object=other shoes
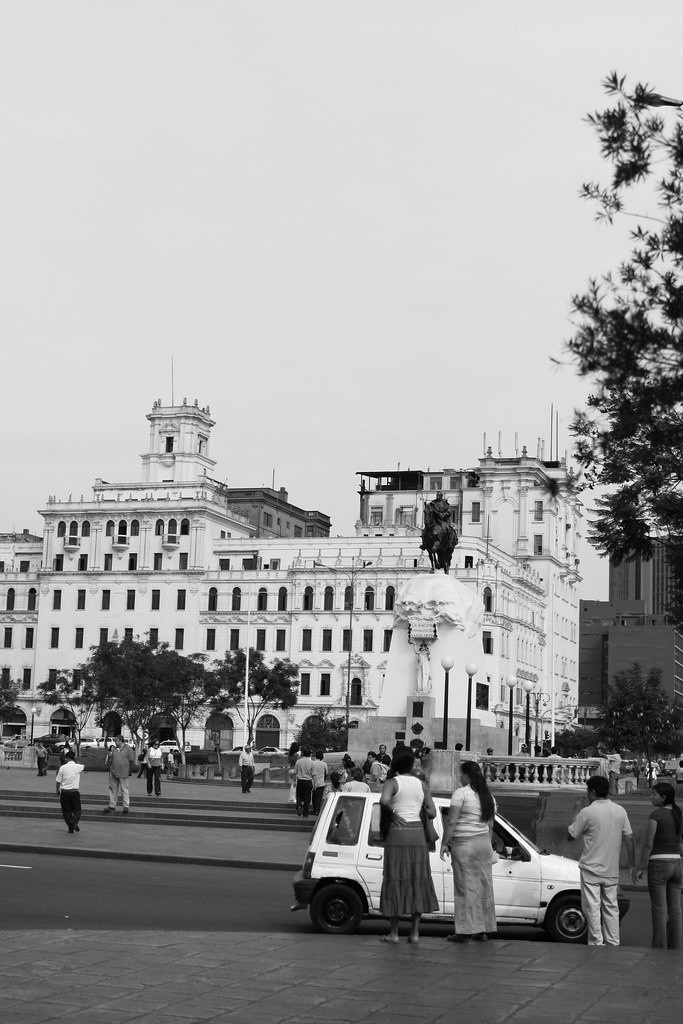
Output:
[104,806,116,811]
[472,934,488,941]
[447,933,468,942]
[71,819,80,831]
[69,830,74,834]
[123,807,129,812]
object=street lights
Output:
[466,663,478,751]
[31,707,36,745]
[506,677,516,756]
[523,680,533,750]
[314,560,373,751]
[441,656,455,750]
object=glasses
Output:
[154,744,159,745]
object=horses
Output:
[422,502,459,575]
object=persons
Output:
[487,748,495,755]
[323,745,392,802]
[455,743,463,751]
[239,745,255,794]
[167,749,174,780]
[36,742,50,776]
[145,739,164,797]
[61,744,70,765]
[288,742,328,817]
[636,782,683,950]
[137,749,148,778]
[56,751,85,833]
[633,767,640,786]
[104,736,135,813]
[567,775,637,947]
[440,761,498,942]
[419,491,451,550]
[513,743,562,783]
[378,746,439,944]
[675,760,683,784]
[105,745,116,780]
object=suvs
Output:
[55,737,98,749]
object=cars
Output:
[253,746,289,757]
[96,737,136,751]
[34,734,71,748]
[220,746,260,755]
[4,734,32,748]
[291,791,632,945]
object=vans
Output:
[159,740,191,752]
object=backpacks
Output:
[138,753,145,761]
[371,762,390,781]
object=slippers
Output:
[379,935,399,943]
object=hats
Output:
[341,756,350,760]
[245,745,250,748]
